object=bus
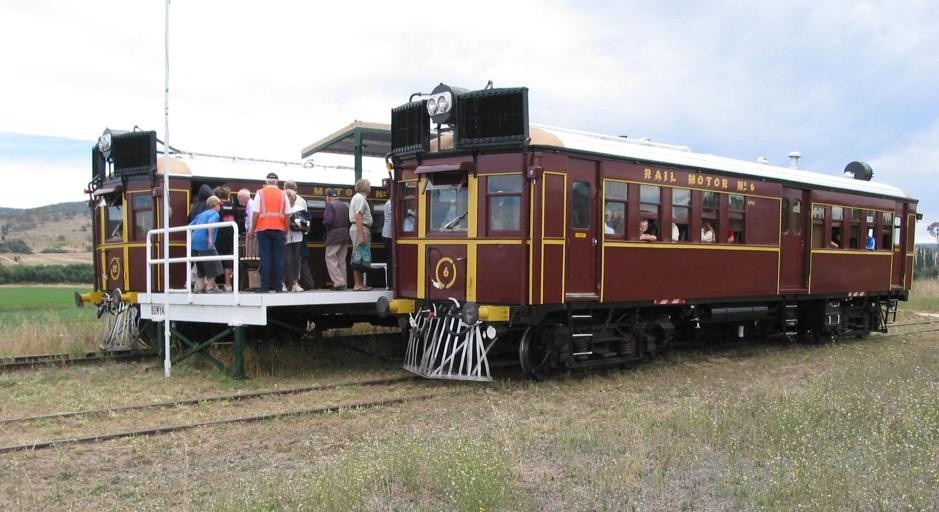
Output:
[390,76,925,384]
[75,125,396,351]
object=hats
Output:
[267,173,278,179]
[206,196,224,207]
[327,189,337,197]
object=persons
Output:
[728,226,736,242]
[831,236,841,249]
[183,170,415,295]
[700,222,716,242]
[865,228,876,250]
[639,219,658,241]
[672,220,680,241]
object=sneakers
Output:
[353,285,373,290]
[331,285,345,291]
[184,282,232,293]
[255,282,304,293]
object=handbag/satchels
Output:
[350,259,370,271]
